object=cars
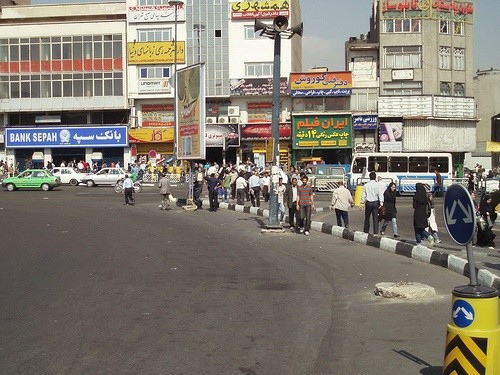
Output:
[80,167,134,188]
[37,167,93,186]
[1,169,62,192]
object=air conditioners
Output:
[129,99,136,128]
[218,117,228,123]
[228,106,240,116]
[239,111,248,124]
[205,117,216,123]
[230,117,238,123]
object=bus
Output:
[348,152,453,195]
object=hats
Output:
[131,163,136,166]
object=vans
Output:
[299,165,346,185]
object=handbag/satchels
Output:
[379,207,385,221]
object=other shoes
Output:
[290,227,294,230]
[429,236,434,244]
[436,240,442,244]
[394,234,399,237]
[132,200,135,205]
[380,230,385,234]
[300,227,304,233]
[304,231,309,235]
[488,246,494,250]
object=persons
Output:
[330,182,355,228]
[413,163,500,249]
[359,172,400,238]
[0,156,316,235]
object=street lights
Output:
[169,0,185,174]
